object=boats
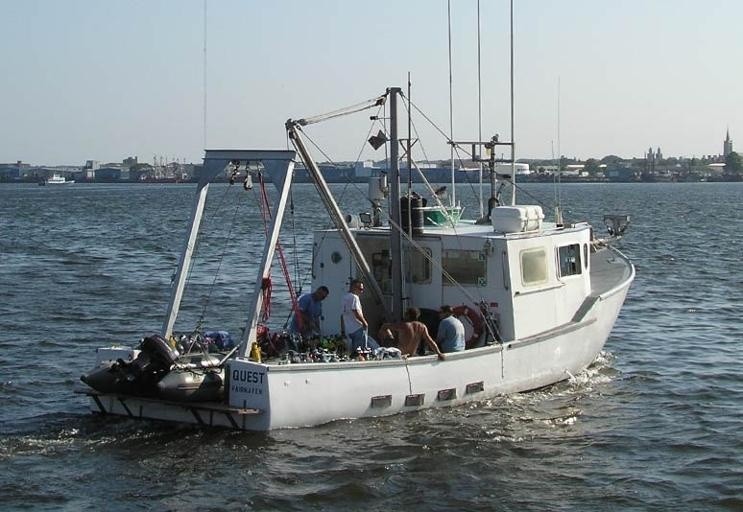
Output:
[38,171,75,187]
[74,86,635,432]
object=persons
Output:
[380,305,447,361]
[431,305,465,353]
[288,285,329,340]
[340,278,368,351]
